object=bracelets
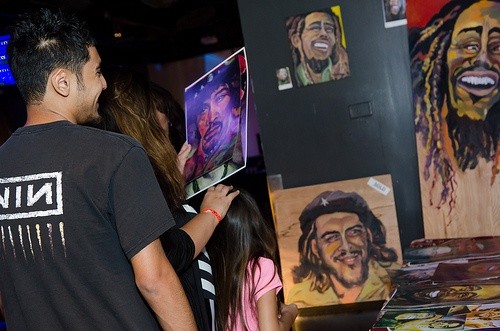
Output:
[201,208,222,222]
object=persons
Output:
[93,69,299,331]
[0,8,198,331]
